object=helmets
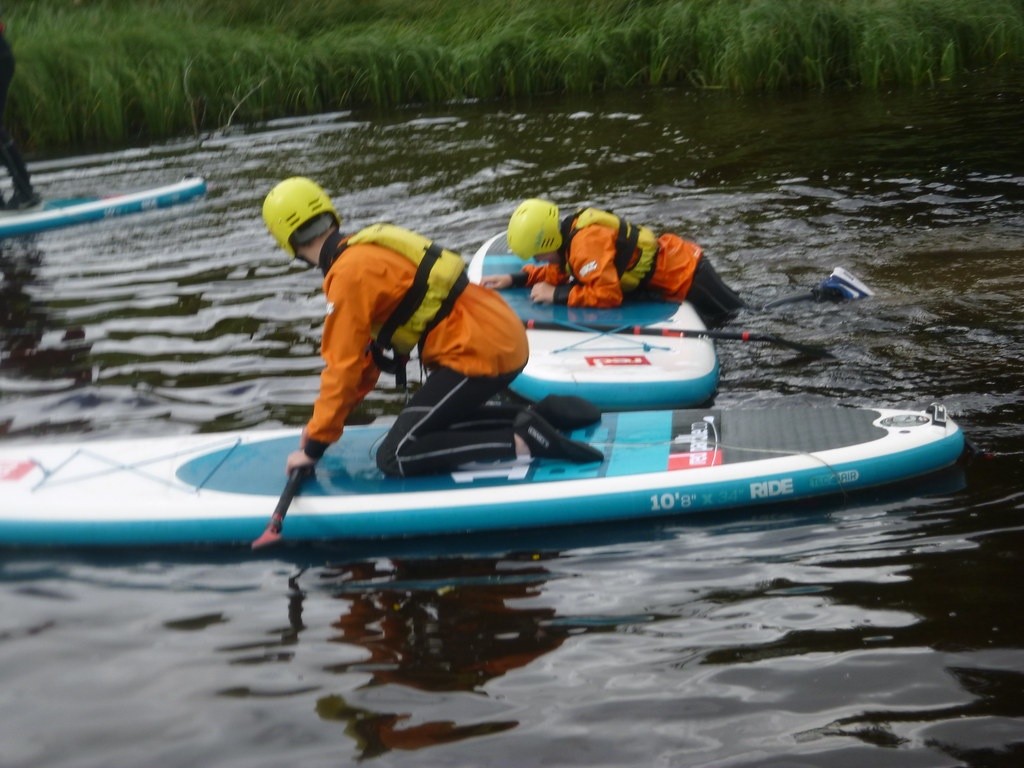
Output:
[507,199,562,261]
[263,176,341,259]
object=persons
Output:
[479,197,875,325]
[260,176,606,479]
[0,17,41,208]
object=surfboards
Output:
[0,401,964,552]
[468,230,720,412]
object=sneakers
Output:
[527,394,600,429]
[514,409,605,463]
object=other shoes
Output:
[823,267,875,300]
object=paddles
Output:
[249,465,301,552]
[522,318,819,352]
